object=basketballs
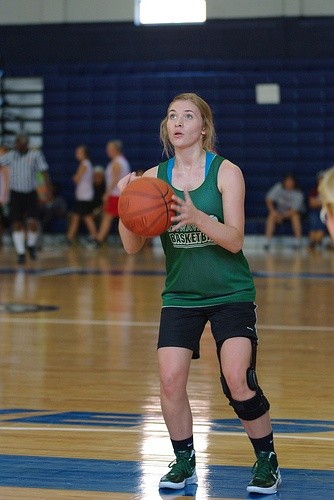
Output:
[118,177,178,237]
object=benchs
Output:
[0,58,334,232]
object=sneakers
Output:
[159,450,198,489]
[247,451,282,494]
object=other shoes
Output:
[87,238,101,247]
[56,239,71,248]
[28,252,37,260]
[16,256,26,264]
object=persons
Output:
[63,138,130,248]
[0,134,62,265]
[263,176,305,252]
[306,167,334,262]
[118,93,282,494]
